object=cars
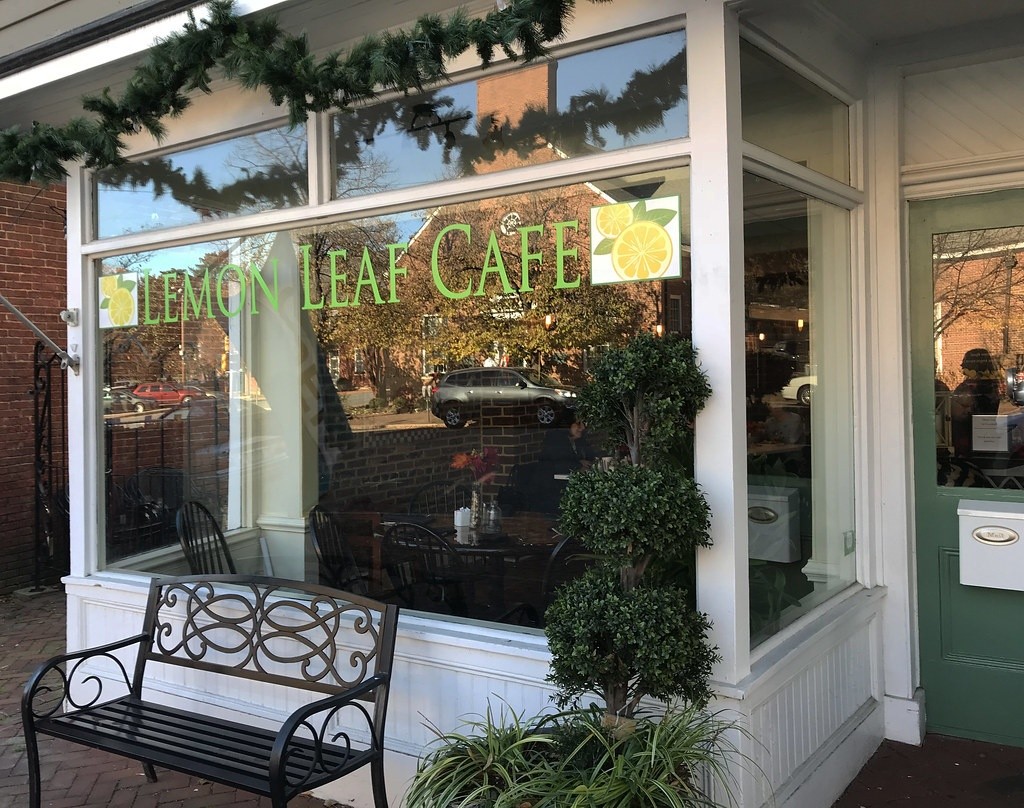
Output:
[782,375,817,406]
[332,376,352,392]
[102,378,270,427]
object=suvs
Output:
[1005,353,1024,407]
[431,367,586,429]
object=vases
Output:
[468,482,482,530]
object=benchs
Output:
[20,575,406,808]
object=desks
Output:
[747,443,805,478]
[380,510,569,621]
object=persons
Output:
[529,407,601,513]
[748,382,806,479]
[950,348,1000,488]
[933,358,952,487]
[937,379,992,488]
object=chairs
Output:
[304,463,583,620]
[175,499,239,576]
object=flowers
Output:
[449,448,499,488]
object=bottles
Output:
[490,501,502,533]
[480,503,489,533]
